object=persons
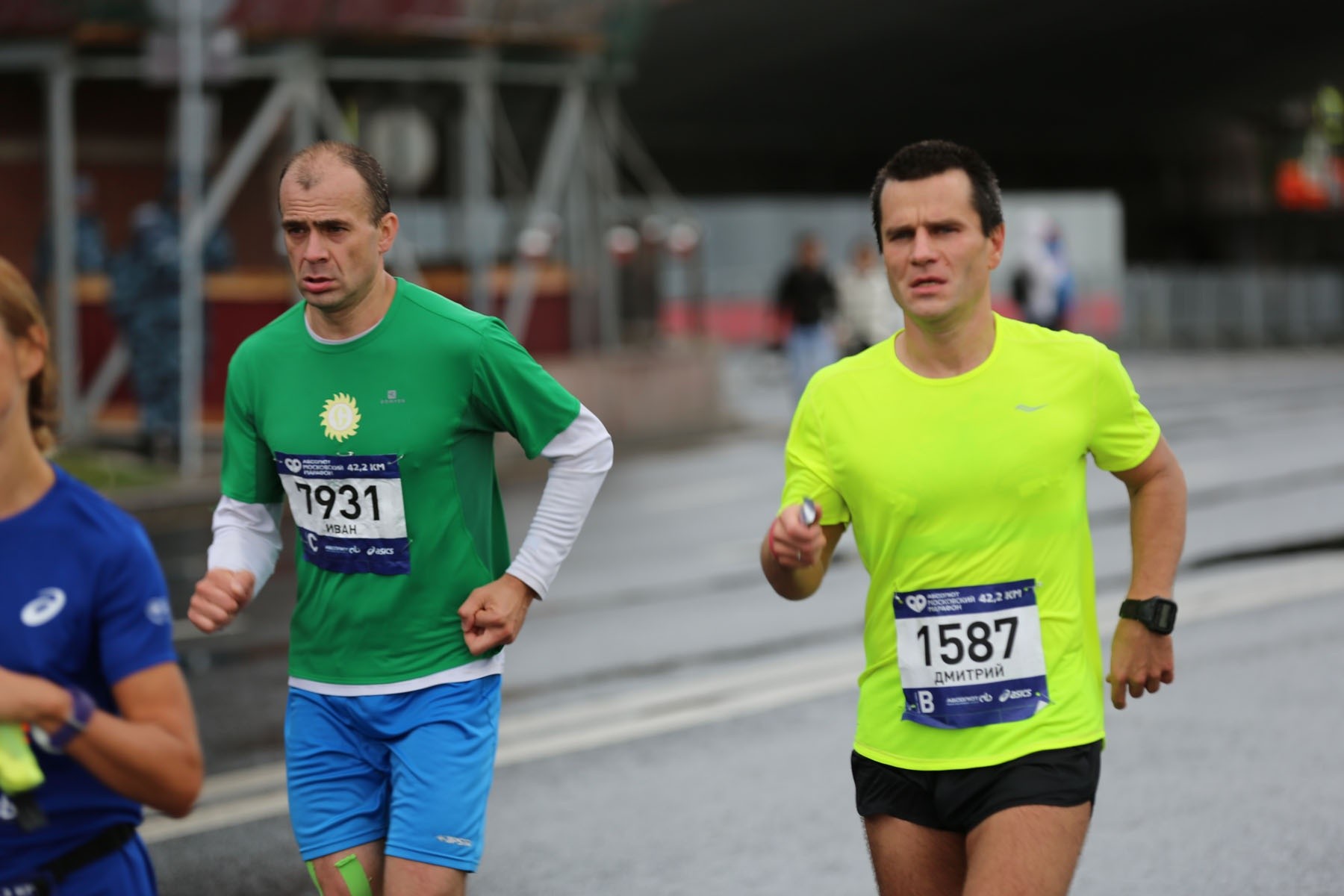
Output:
[20,166,207,467]
[186,143,615,895]
[760,138,1186,896]
[0,250,203,896]
[771,202,1070,406]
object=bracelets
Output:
[769,518,778,562]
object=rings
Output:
[797,549,805,565]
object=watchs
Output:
[32,686,93,757]
[1119,596,1178,637]
